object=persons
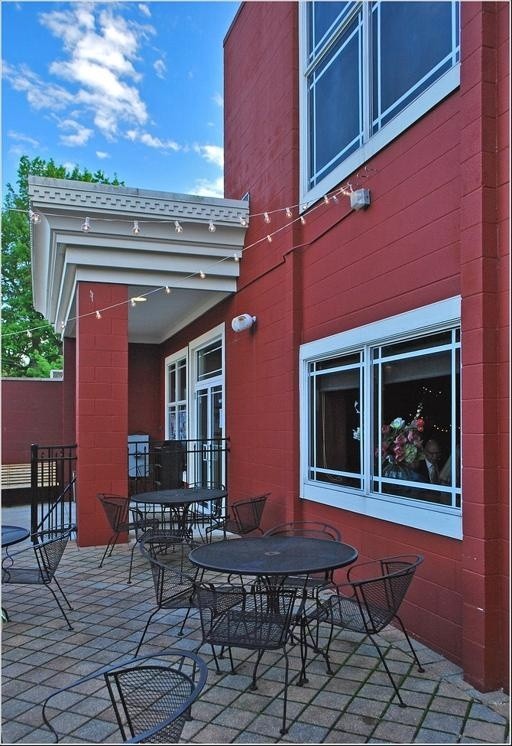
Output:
[415,438,445,502]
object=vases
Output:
[382,461,414,493]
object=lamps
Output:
[231,314,257,334]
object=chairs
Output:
[314,553,425,709]
[41,650,208,745]
[206,491,272,553]
[1,523,76,631]
[95,492,160,583]
[252,519,343,644]
[131,534,246,675]
[186,580,333,735]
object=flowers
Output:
[350,401,428,468]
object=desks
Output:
[131,488,229,551]
[0,524,31,622]
[189,535,360,691]
